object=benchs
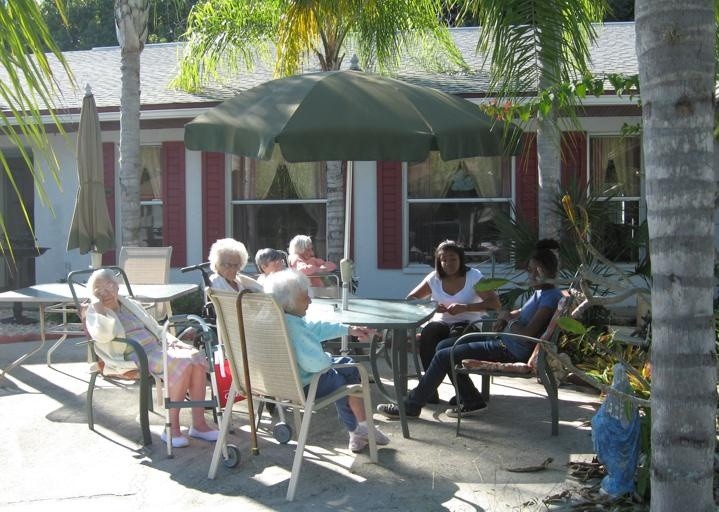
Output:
[605,295,651,347]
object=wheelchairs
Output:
[273,248,364,363]
[179,260,221,410]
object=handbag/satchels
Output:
[212,359,247,408]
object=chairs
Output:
[382,251,500,402]
[77,246,177,364]
[204,286,378,502]
[450,289,571,437]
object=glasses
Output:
[223,263,240,269]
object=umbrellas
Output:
[67,83,116,275]
[183,52,526,359]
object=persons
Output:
[206,236,275,413]
[286,234,358,298]
[265,267,390,451]
[419,194,486,263]
[401,239,501,404]
[376,237,566,419]
[253,248,284,285]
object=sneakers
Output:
[446,402,488,417]
[189,424,220,441]
[349,422,389,452]
[160,430,189,447]
[377,404,420,419]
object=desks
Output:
[302,296,439,438]
[0,283,199,387]
